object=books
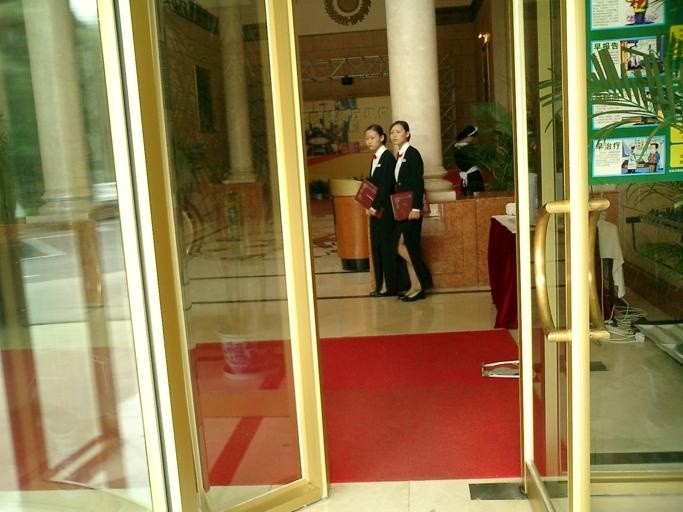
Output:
[389,189,431,222]
[355,178,385,219]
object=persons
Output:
[453,126,485,196]
[628,147,637,173]
[631,0,649,25]
[389,120,433,301]
[628,45,642,70]
[364,125,397,297]
[648,143,660,172]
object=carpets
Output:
[0,328,573,491]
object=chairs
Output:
[167,130,268,262]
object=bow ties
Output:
[397,152,402,160]
[372,154,376,159]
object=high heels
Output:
[370,283,425,304]
